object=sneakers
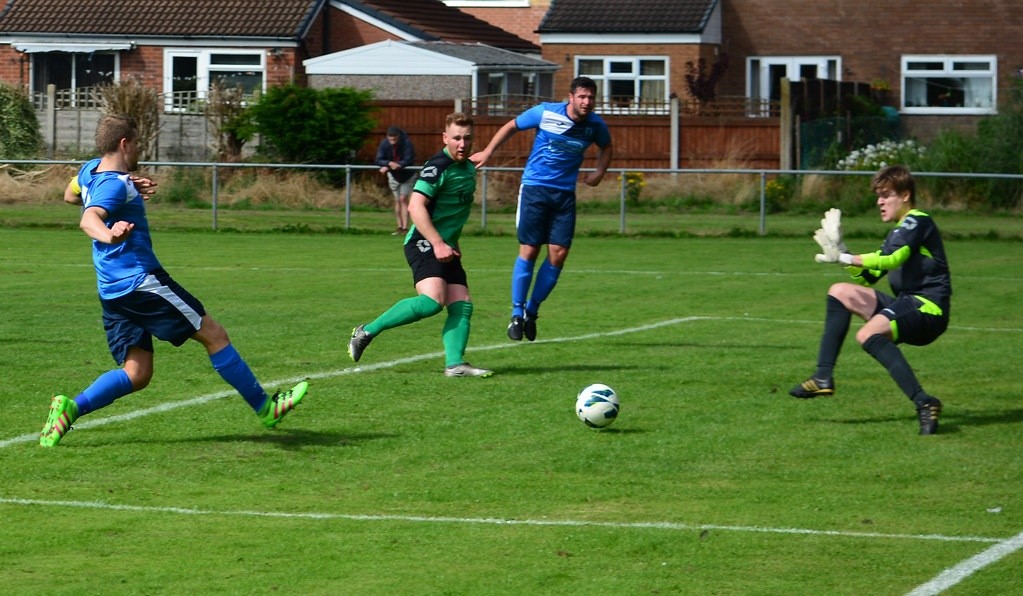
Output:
[347,325,372,363]
[38,394,76,447]
[444,362,495,378]
[790,376,835,398]
[916,397,942,434]
[507,317,522,339]
[260,381,307,428]
[524,308,538,341]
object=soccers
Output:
[575,383,619,428]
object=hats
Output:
[388,126,398,135]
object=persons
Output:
[345,111,496,378]
[789,167,954,435]
[467,78,613,342]
[377,126,419,235]
[39,113,310,452]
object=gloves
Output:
[821,207,848,254]
[813,228,840,264]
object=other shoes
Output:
[391,227,407,237]
